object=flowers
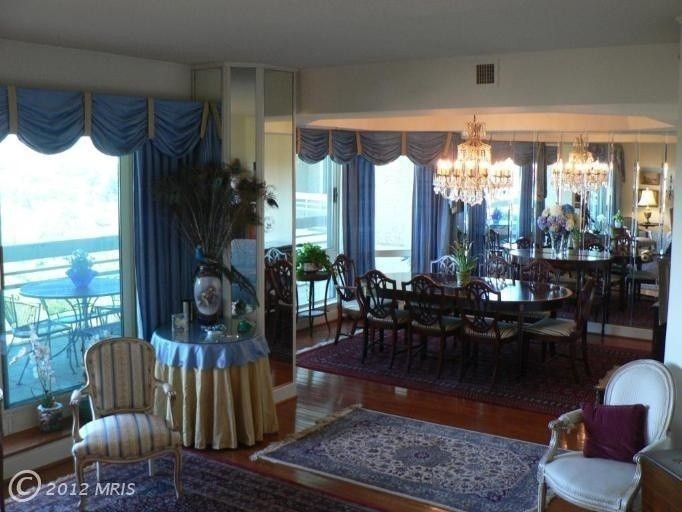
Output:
[537,202,578,249]
[64,249,96,272]
[9,324,57,408]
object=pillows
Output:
[578,401,649,465]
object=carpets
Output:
[4,448,385,512]
[249,404,578,512]
[297,317,657,416]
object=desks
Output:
[633,448,682,512]
[155,322,263,450]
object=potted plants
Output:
[613,209,624,229]
[295,243,332,281]
[437,223,484,288]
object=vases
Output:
[193,263,222,329]
[37,402,64,434]
[65,269,98,289]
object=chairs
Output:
[485,217,667,325]
[536,359,676,512]
[4,276,121,385]
[68,337,184,512]
[330,254,605,389]
[264,248,292,349]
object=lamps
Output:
[432,112,513,207]
[638,187,659,224]
[552,134,609,196]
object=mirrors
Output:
[191,62,296,405]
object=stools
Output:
[296,269,331,338]
